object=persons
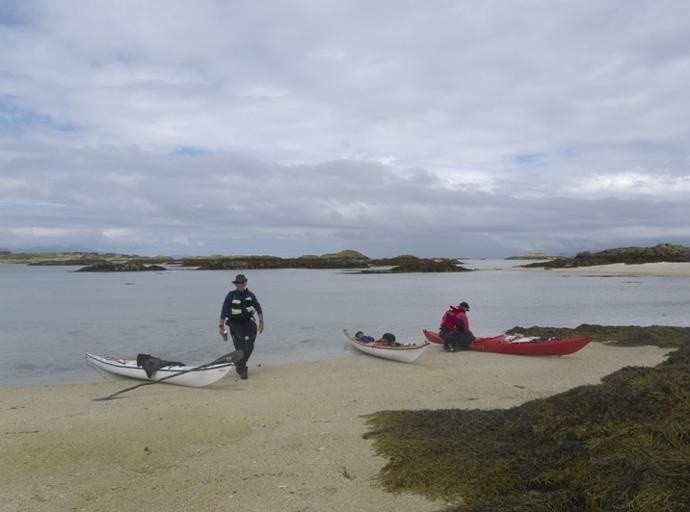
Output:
[217,274,265,379]
[439,301,470,352]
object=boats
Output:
[86,352,235,389]
[342,323,593,364]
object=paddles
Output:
[111,350,243,397]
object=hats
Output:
[232,275,248,283]
[460,302,469,309]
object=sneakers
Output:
[236,365,249,379]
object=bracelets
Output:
[220,325,224,328]
[259,320,263,323]
